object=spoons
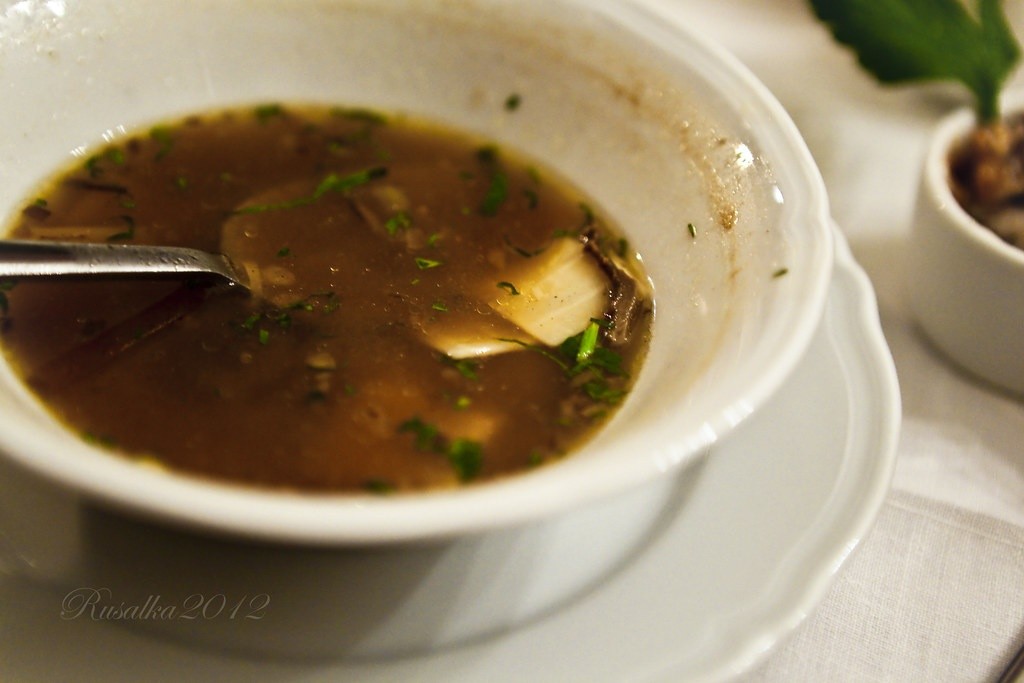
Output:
[0,176,356,302]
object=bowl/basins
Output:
[0,0,837,546]
[911,90,1024,404]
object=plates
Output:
[1,210,904,681]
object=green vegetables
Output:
[28,97,631,491]
